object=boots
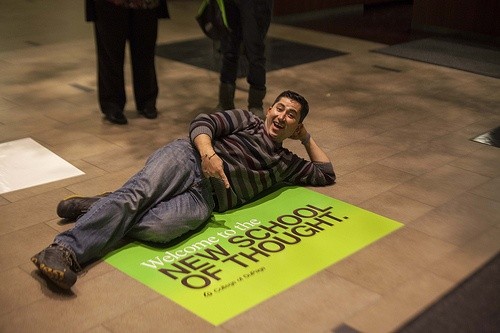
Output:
[248,86,267,120]
[220,82,235,111]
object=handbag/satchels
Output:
[195,0,242,45]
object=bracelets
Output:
[201,153,215,160]
[302,135,309,144]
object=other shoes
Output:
[106,112,127,124]
[137,107,156,118]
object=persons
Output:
[218,0,272,111]
[84,0,171,124]
[31,90,336,294]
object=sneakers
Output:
[57,191,113,220]
[31,244,78,290]
